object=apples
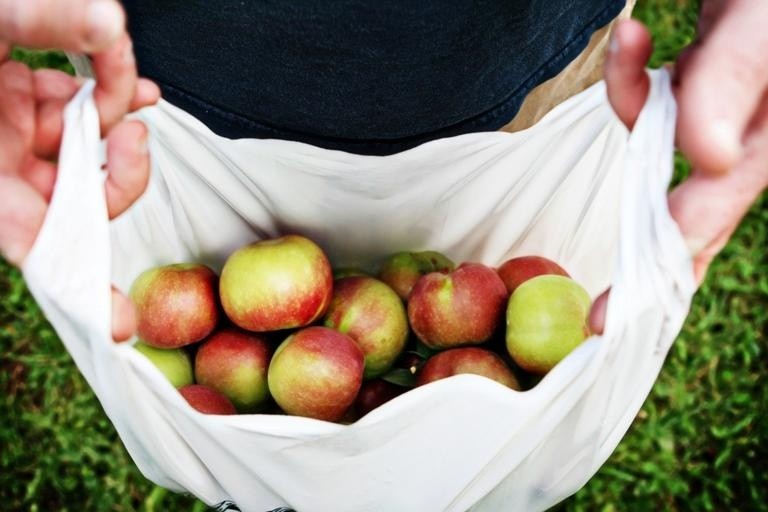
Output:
[126,233,594,428]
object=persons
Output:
[0,0,767,345]
